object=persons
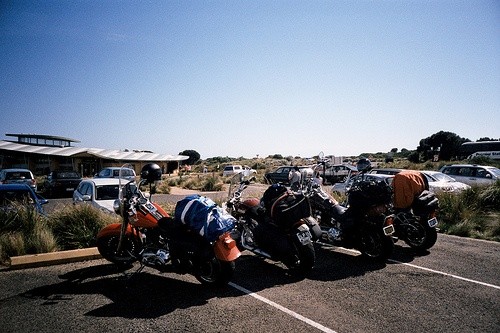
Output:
[288,168,302,192]
[163,164,220,174]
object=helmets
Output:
[356,159,372,173]
[140,163,162,181]
[262,184,287,211]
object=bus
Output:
[461,140,500,161]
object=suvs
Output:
[438,164,500,189]
[264,166,314,185]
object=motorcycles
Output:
[96,162,242,290]
[225,152,441,275]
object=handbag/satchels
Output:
[270,191,310,224]
[343,178,394,211]
[174,193,236,239]
[392,171,429,209]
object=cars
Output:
[43,169,83,198]
[0,184,49,216]
[0,168,38,194]
[330,167,407,201]
[419,171,471,197]
[72,178,150,215]
[94,167,136,185]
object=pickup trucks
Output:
[318,164,359,183]
[223,165,257,178]
